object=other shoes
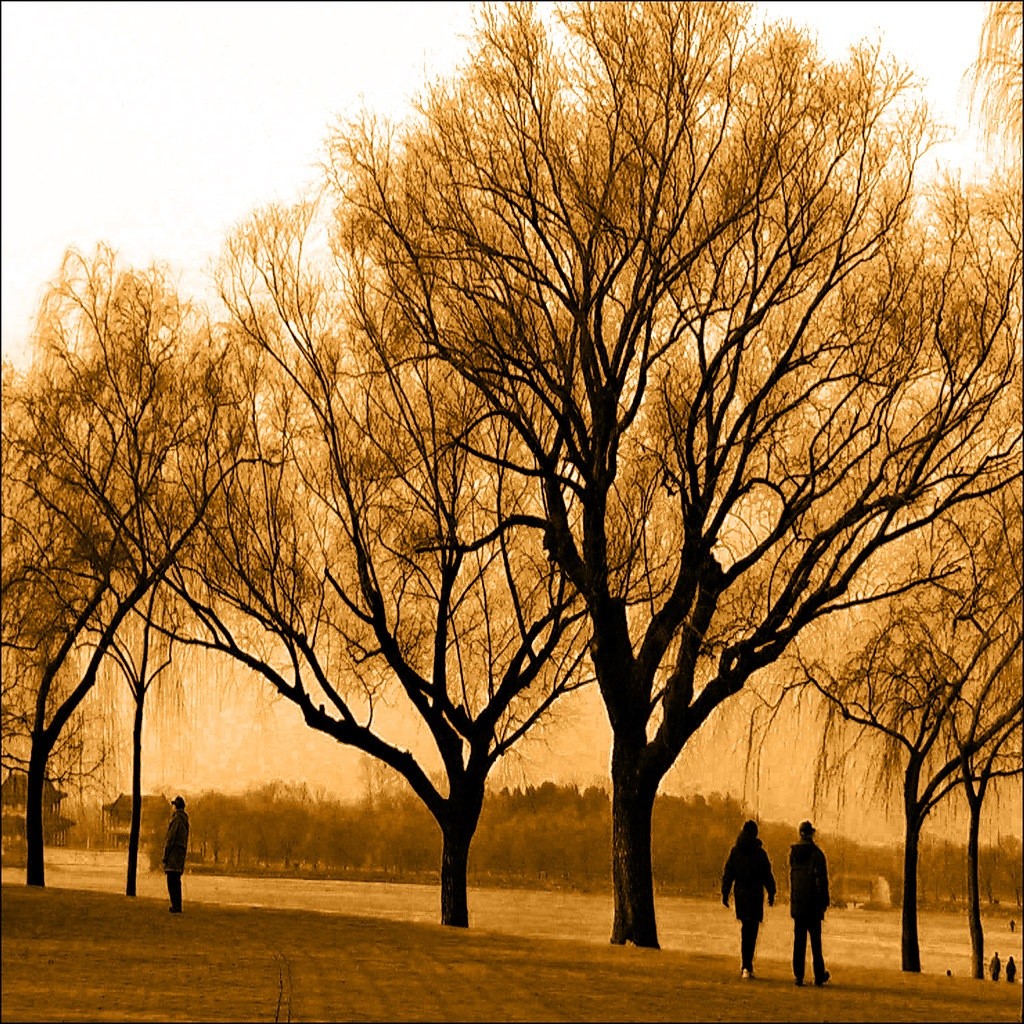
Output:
[169,907,182,913]
[815,971,829,986]
[795,979,803,987]
[742,969,754,979]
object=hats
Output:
[743,820,758,834]
[171,797,186,807]
[799,822,816,837]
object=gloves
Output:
[722,894,729,909]
[768,895,774,907]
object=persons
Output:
[160,795,190,913]
[786,821,832,986]
[1005,956,1017,983]
[1010,918,1016,932]
[990,951,1000,982]
[719,819,776,980]
